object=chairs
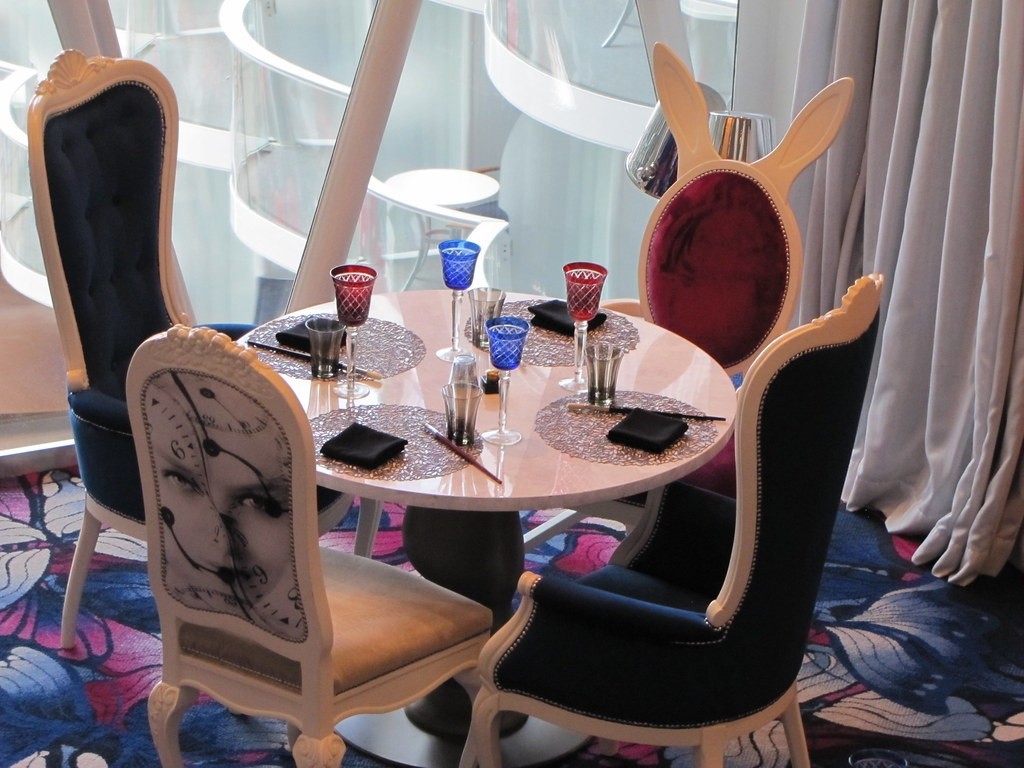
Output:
[29,48,184,651]
[126,328,491,767]
[521,42,854,582]
[476,274,881,765]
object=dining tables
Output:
[234,289,737,765]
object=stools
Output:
[384,168,497,290]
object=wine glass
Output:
[436,240,480,361]
[479,315,529,444]
[330,264,378,397]
[557,261,608,393]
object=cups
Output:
[304,316,345,378]
[468,286,506,349]
[586,341,622,404]
[441,382,484,448]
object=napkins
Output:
[527,300,606,334]
[321,422,407,468]
[277,316,347,353]
[608,409,687,453]
